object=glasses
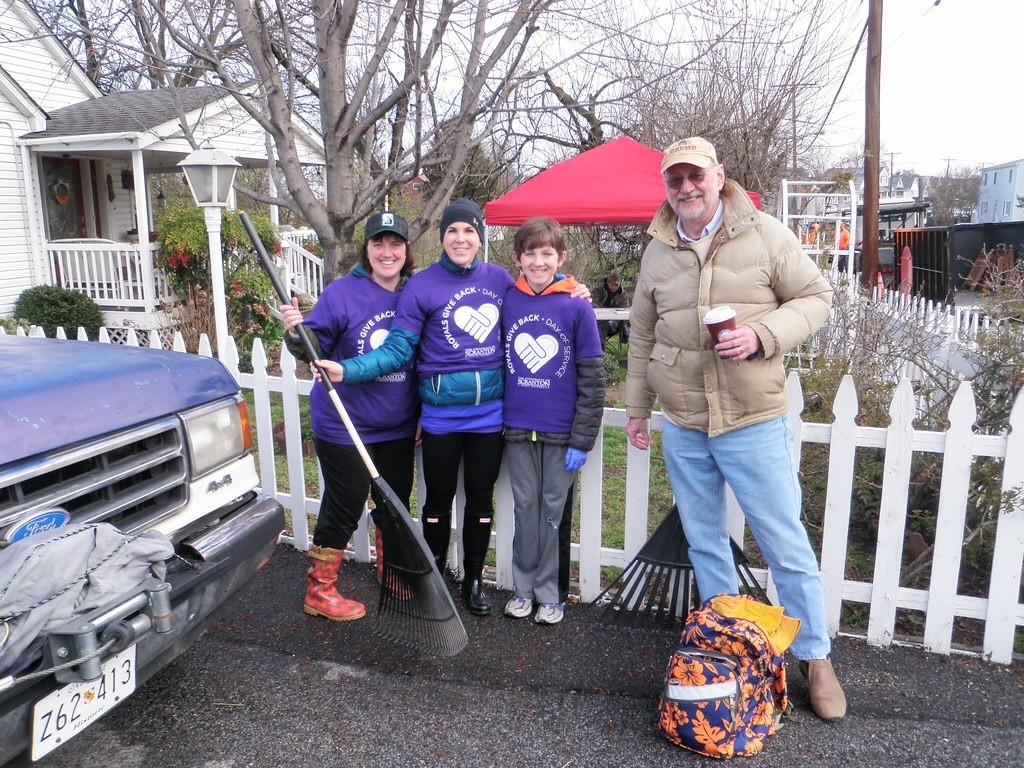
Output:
[665,167,707,190]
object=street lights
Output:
[175,141,242,361]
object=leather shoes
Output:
[799,656,847,722]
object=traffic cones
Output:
[877,272,885,299]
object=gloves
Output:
[563,446,587,472]
[710,596,801,657]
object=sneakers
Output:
[534,602,565,624]
[504,596,536,618]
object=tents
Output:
[485,135,762,264]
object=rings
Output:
[739,347,743,353]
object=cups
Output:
[703,306,737,360]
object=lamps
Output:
[157,162,166,205]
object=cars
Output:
[878,213,905,222]
[838,246,894,290]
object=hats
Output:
[660,136,719,176]
[440,198,484,245]
[364,211,408,241]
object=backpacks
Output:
[654,594,788,760]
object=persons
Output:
[828,222,850,274]
[801,223,815,244]
[591,271,630,353]
[280,210,416,623]
[624,135,846,722]
[500,216,605,624]
[310,198,593,616]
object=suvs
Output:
[0,335,285,766]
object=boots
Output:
[461,511,494,615]
[304,544,365,621]
[421,506,453,576]
[375,525,414,600]
